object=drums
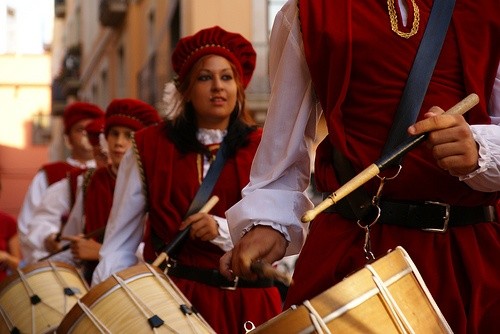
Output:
[0,260,93,334]
[244,246,455,334]
[54,261,218,334]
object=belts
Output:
[164,262,275,291]
[320,190,496,233]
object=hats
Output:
[171,26,256,92]
[103,97,163,131]
[86,116,106,133]
[61,100,105,131]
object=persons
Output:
[59,96,162,288]
[22,118,109,262]
[220,0,500,334]
[17,102,106,266]
[91,25,299,334]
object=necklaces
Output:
[387,0,420,38]
